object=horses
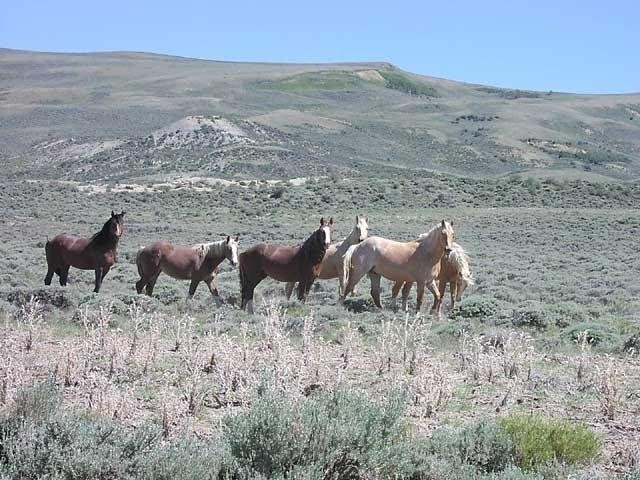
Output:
[340,218,455,315]
[285,216,369,300]
[44,209,126,293]
[391,242,476,313]
[239,216,334,315]
[136,235,239,301]
